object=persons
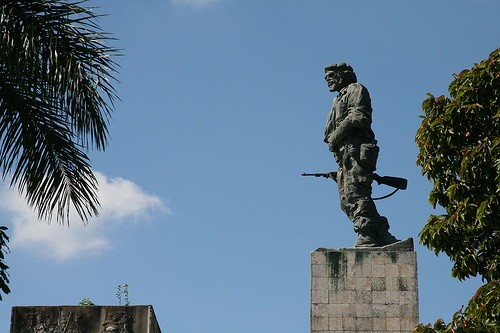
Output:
[323,63,401,247]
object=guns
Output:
[301,170,408,190]
